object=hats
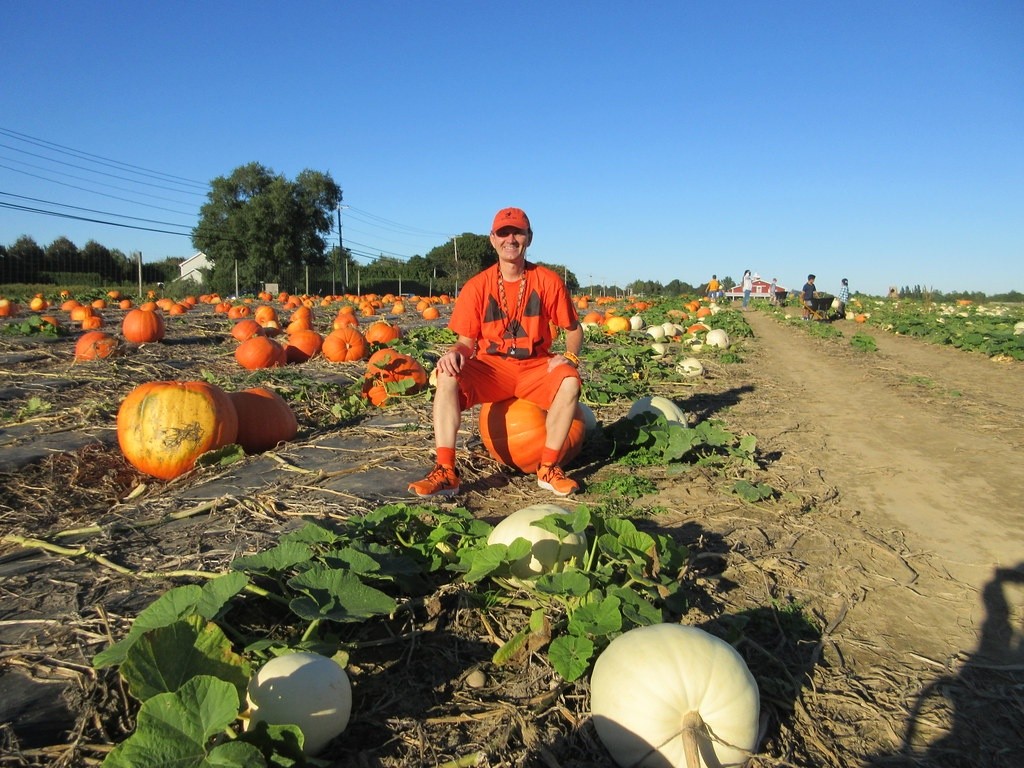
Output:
[492,207,530,230]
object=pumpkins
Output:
[1,287,762,768]
[831,298,871,324]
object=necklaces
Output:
[496,260,527,355]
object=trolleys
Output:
[800,296,835,323]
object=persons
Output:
[403,206,586,501]
[768,277,778,305]
[720,282,727,297]
[886,288,896,298]
[741,269,753,311]
[799,274,819,321]
[836,277,850,320]
[705,274,718,302]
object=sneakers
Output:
[408,464,460,497]
[535,462,580,495]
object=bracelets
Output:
[563,351,580,368]
